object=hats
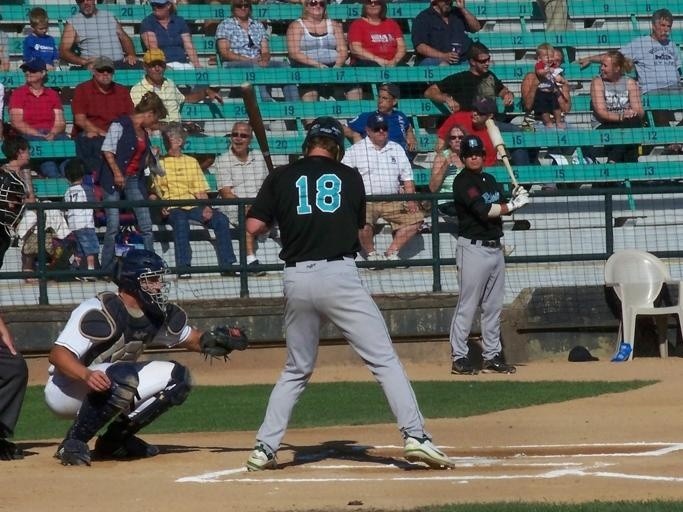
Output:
[380,83,399,109]
[19,58,47,70]
[93,55,115,70]
[473,97,497,114]
[568,345,598,361]
[367,113,388,128]
[149,0,168,4]
[143,48,165,63]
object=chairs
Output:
[604,250,683,360]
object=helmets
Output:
[114,250,171,329]
[460,134,483,155]
[1,169,30,239]
[303,116,345,161]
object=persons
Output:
[533,0,576,63]
[571,8,683,155]
[246,116,456,472]
[0,1,535,283]
[590,50,644,164]
[1,318,29,460]
[522,43,584,189]
[43,248,249,466]
[449,135,530,375]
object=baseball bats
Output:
[485,118,519,188]
[240,82,274,174]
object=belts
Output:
[286,257,343,266]
[472,240,500,249]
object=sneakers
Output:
[221,260,265,277]
[503,245,516,256]
[367,250,409,270]
[178,274,192,278]
[0,438,23,460]
[246,443,278,471]
[482,357,516,374]
[404,434,455,470]
[95,433,146,460]
[54,439,91,467]
[452,358,478,376]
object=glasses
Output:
[235,3,249,8]
[372,127,387,132]
[308,0,326,6]
[232,132,250,138]
[475,56,491,64]
[449,135,463,140]
[366,0,381,5]
[96,68,112,73]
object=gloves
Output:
[506,186,529,212]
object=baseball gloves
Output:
[199,325,247,356]
